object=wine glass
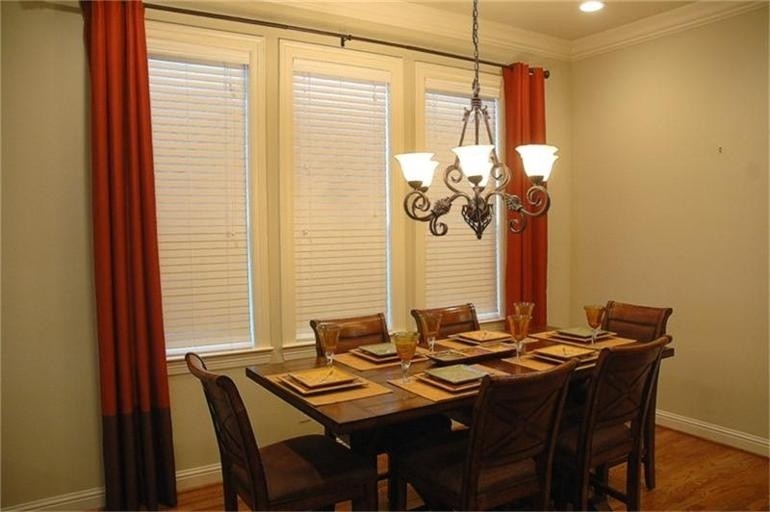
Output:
[505,301,536,363]
[583,304,607,347]
[391,332,421,384]
[315,322,345,377]
[419,310,443,352]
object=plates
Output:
[348,342,422,366]
[528,344,603,366]
[445,329,515,346]
[412,364,489,392]
[549,326,619,342]
[273,366,370,395]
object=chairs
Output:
[562,332,673,512]
[598,299,675,490]
[183,351,378,512]
[303,312,393,446]
[409,303,480,342]
[388,356,582,510]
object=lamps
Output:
[392,3,561,240]
